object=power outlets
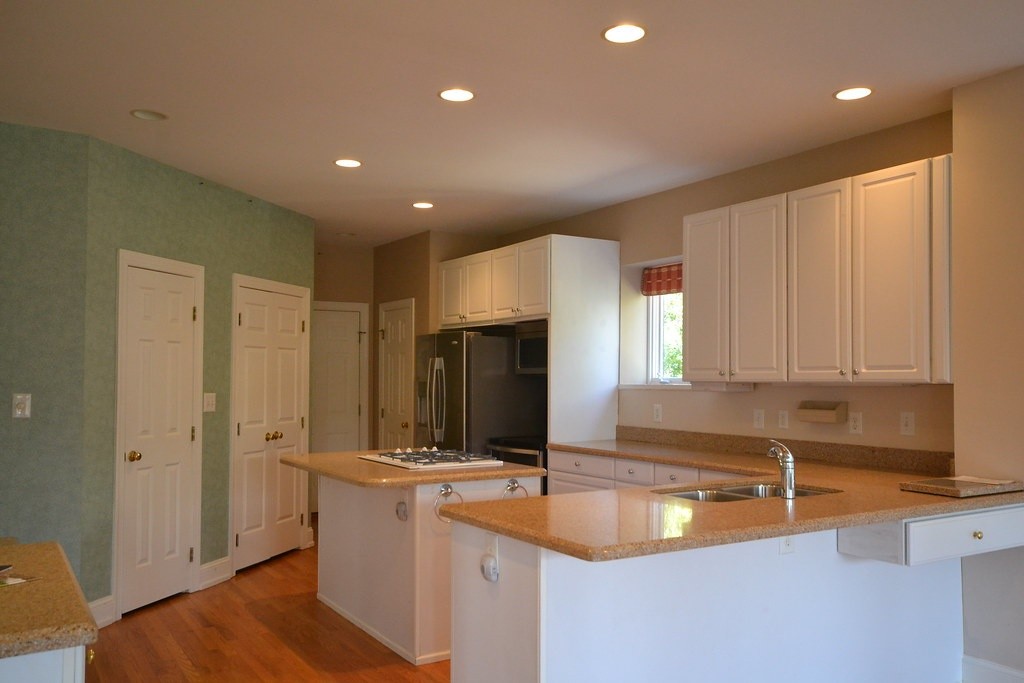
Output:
[753,408,765,430]
[779,410,789,429]
[652,402,662,424]
[899,409,916,437]
[849,413,865,434]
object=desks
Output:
[487,432,548,490]
[279,450,546,666]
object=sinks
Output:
[720,484,830,498]
[659,489,756,503]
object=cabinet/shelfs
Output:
[835,501,1022,566]
[492,237,550,323]
[548,452,739,495]
[681,191,786,381]
[786,157,950,384]
[437,250,491,327]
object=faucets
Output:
[767,439,796,499]
